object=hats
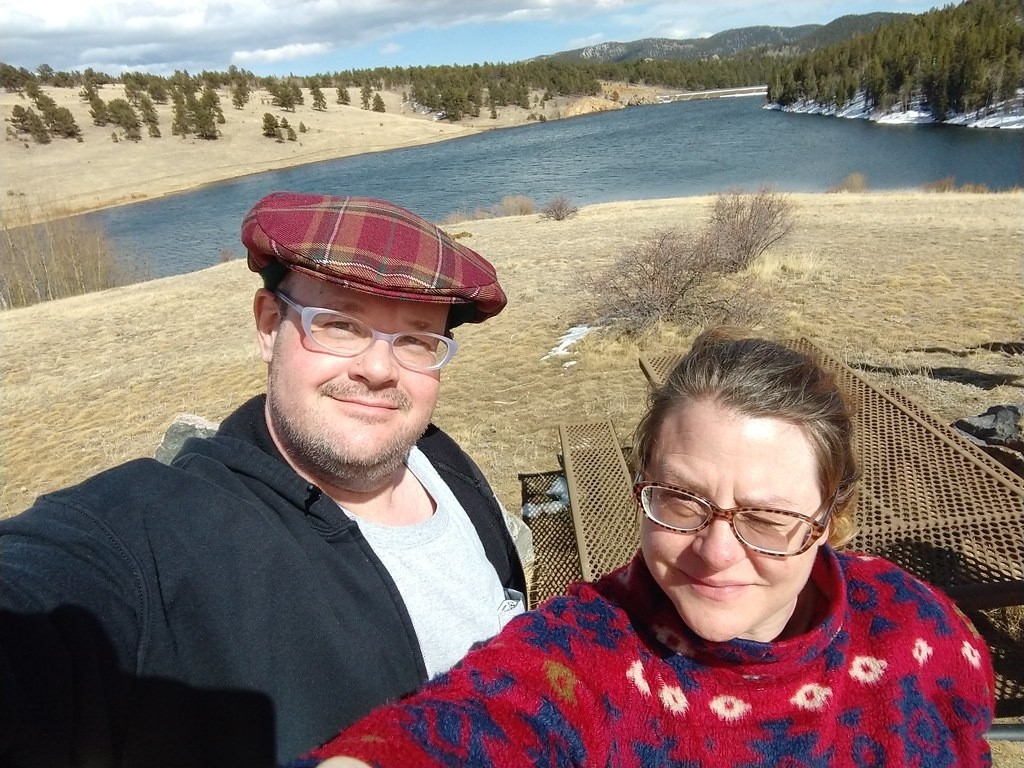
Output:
[240,192,507,330]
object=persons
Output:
[1,189,533,768]
[290,330,998,768]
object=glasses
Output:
[632,457,839,556]
[274,290,458,371]
